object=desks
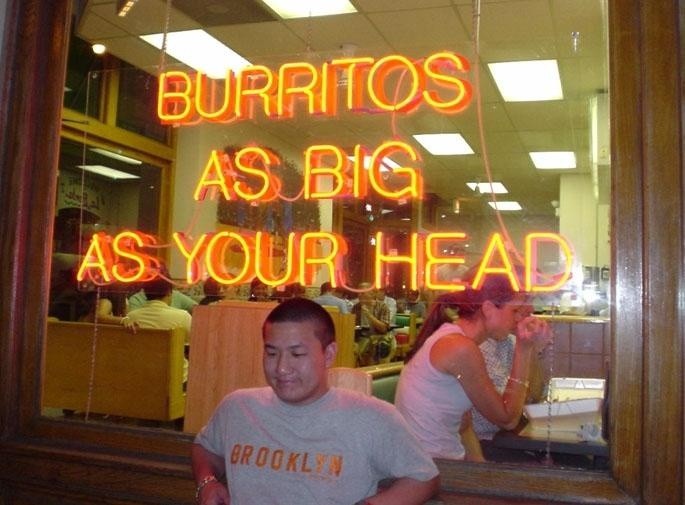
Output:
[493,413,610,458]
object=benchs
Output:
[42,319,186,422]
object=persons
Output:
[462,248,554,467]
[311,282,425,367]
[392,261,543,466]
[68,274,305,393]
[188,295,444,505]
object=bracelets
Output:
[506,376,530,389]
[120,316,129,324]
[193,474,217,499]
